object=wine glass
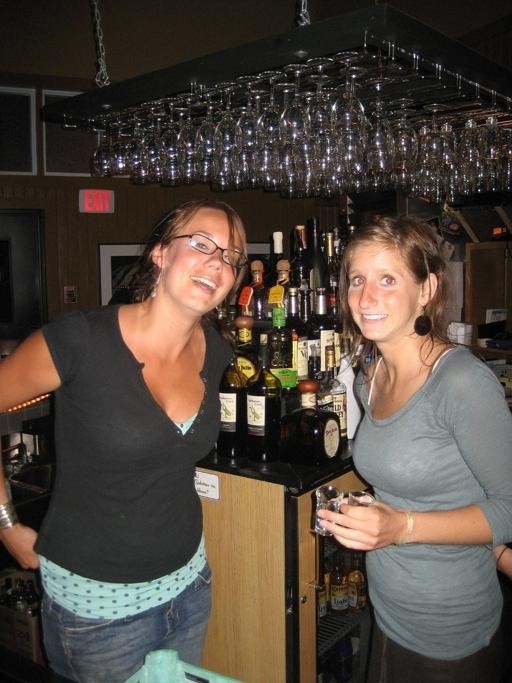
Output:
[87,49,511,206]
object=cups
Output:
[311,483,377,540]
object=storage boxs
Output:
[0,603,49,670]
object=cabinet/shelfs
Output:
[195,440,379,682]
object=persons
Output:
[0,198,248,683]
[315,213,512,683]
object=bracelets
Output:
[0,501,20,529]
[496,546,508,568]
[399,508,413,547]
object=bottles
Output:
[316,628,361,682]
[218,216,384,465]
[1,575,45,616]
[317,544,370,618]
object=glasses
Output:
[162,231,249,269]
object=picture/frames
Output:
[97,240,273,311]
